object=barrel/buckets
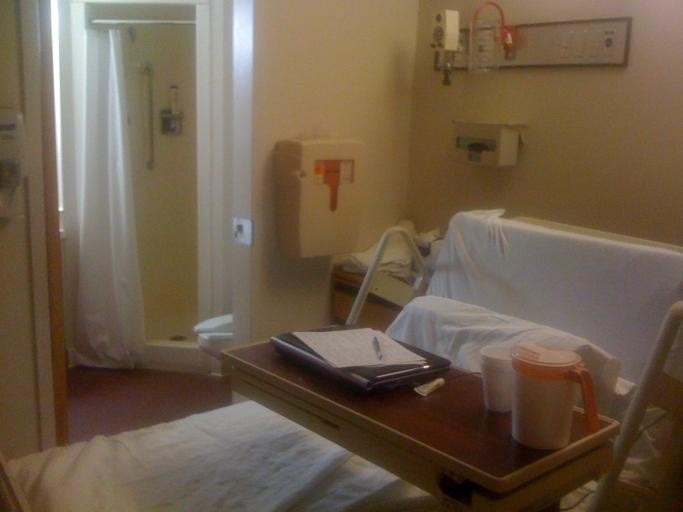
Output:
[511,340,596,448]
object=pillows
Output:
[384,294,617,416]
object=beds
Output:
[0,208,683,512]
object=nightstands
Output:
[330,262,430,332]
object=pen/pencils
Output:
[373,336,383,359]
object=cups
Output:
[478,345,597,450]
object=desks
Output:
[216,322,621,511]
